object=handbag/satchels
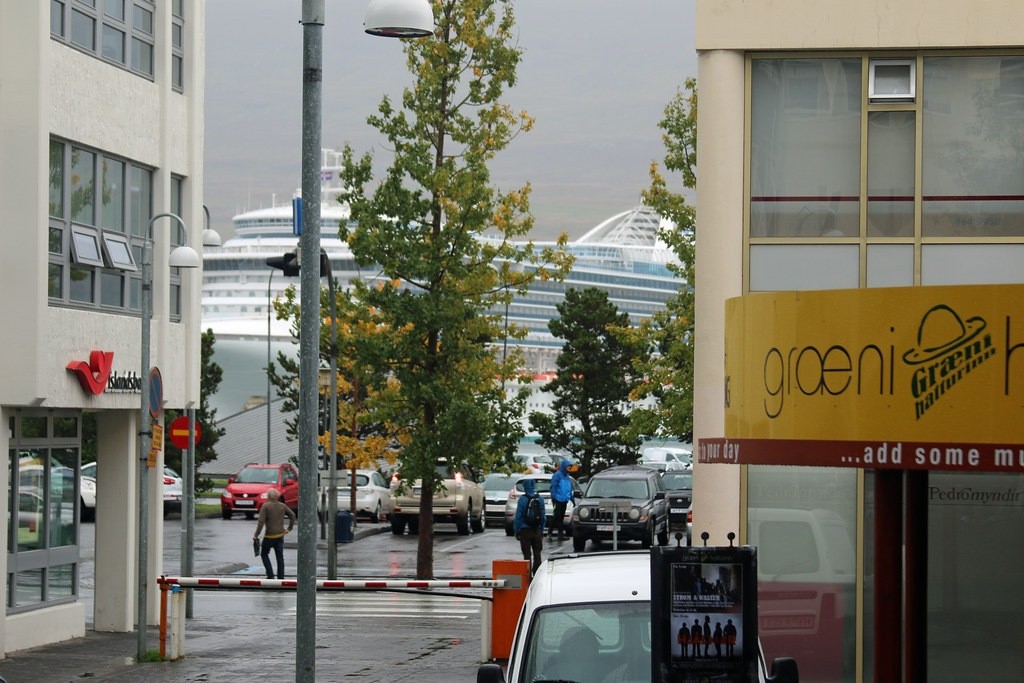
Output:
[254,537,260,556]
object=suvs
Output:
[389,456,487,535]
[221,463,299,520]
[571,468,670,552]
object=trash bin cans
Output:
[335,509,355,543]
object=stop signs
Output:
[169,416,201,448]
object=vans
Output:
[645,447,693,471]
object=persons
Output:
[543,626,601,683]
[690,573,727,602]
[678,615,736,657]
[546,459,576,542]
[255,488,296,579]
[513,479,547,576]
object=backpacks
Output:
[522,494,541,527]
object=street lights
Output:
[180,204,222,578]
[136,212,200,664]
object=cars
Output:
[483,473,523,521]
[8,485,74,549]
[660,471,692,528]
[505,474,584,537]
[317,469,392,524]
[81,461,182,518]
[749,507,870,619]
[515,453,555,474]
[476,549,800,683]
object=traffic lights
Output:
[266,253,327,276]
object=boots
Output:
[547,527,554,541]
[558,529,570,541]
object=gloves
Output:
[516,532,521,540]
[541,531,544,538]
[571,499,576,507]
[552,498,556,506]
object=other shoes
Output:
[266,575,274,579]
